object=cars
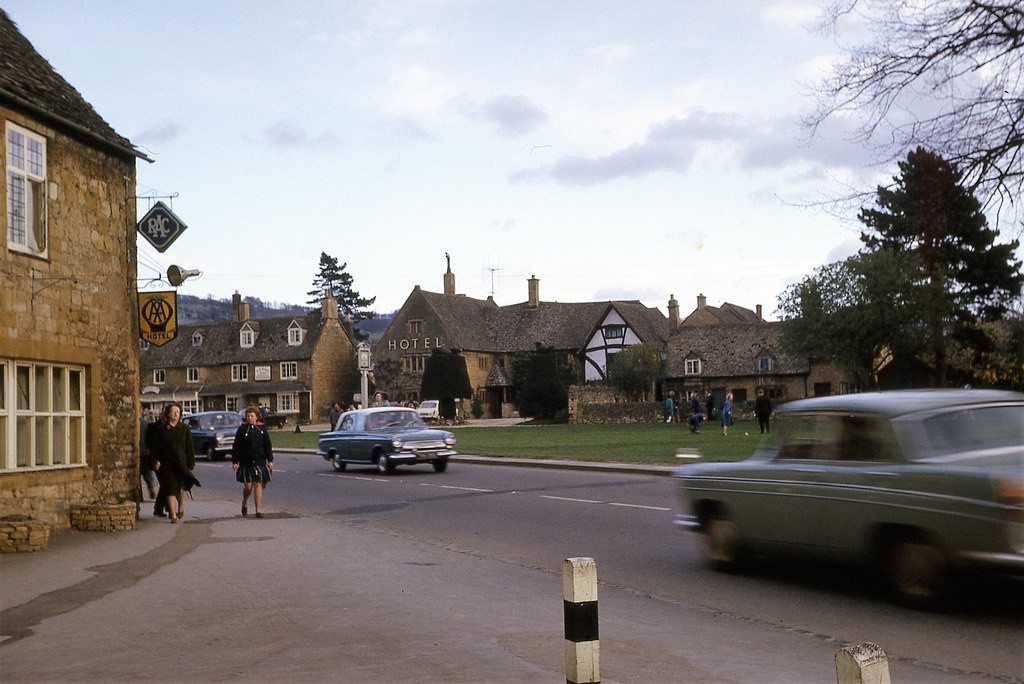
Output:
[317,407,458,479]
[181,410,244,460]
[261,405,288,429]
[674,385,1023,607]
[417,400,445,423]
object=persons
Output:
[329,402,345,432]
[371,391,383,408]
[669,391,680,423]
[207,403,236,427]
[753,388,775,436]
[705,389,715,420]
[152,401,196,524]
[721,392,734,437]
[238,402,272,424]
[346,401,357,412]
[407,398,414,409]
[380,392,390,408]
[664,394,674,422]
[394,398,403,406]
[136,403,157,502]
[688,392,700,418]
[141,406,161,422]
[146,405,175,520]
[230,406,274,517]
[356,401,362,411]
[688,413,707,434]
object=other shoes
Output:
[242,503,247,517]
[173,510,184,523]
[256,513,264,518]
